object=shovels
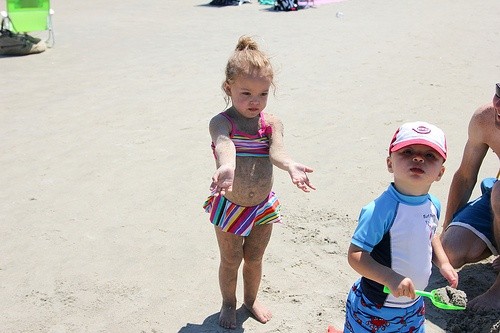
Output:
[384,287,468,311]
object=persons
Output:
[203,37,316,329]
[440,83,500,313]
[329,121,459,333]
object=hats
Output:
[388,121,448,163]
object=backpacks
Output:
[0,28,46,57]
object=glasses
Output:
[495,83,500,97]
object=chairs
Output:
[0,0,55,48]
